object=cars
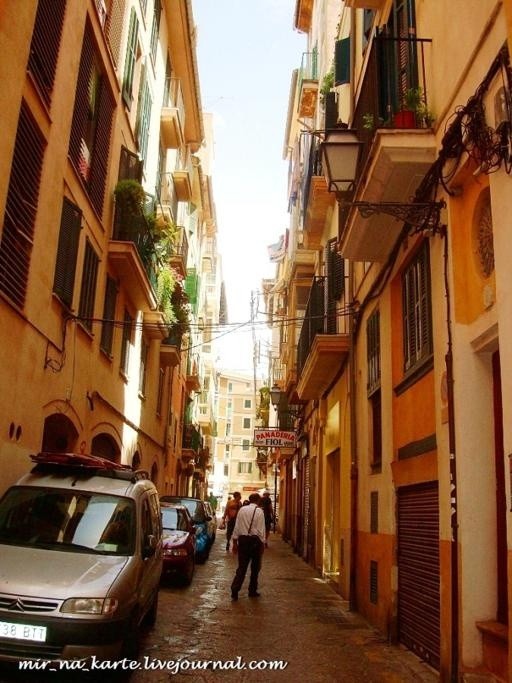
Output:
[159,495,218,586]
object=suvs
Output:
[0,451,166,682]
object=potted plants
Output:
[114,178,191,349]
[389,86,424,130]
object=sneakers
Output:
[249,591,259,597]
[231,591,238,599]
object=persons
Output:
[219,488,275,601]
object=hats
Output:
[263,491,270,496]
